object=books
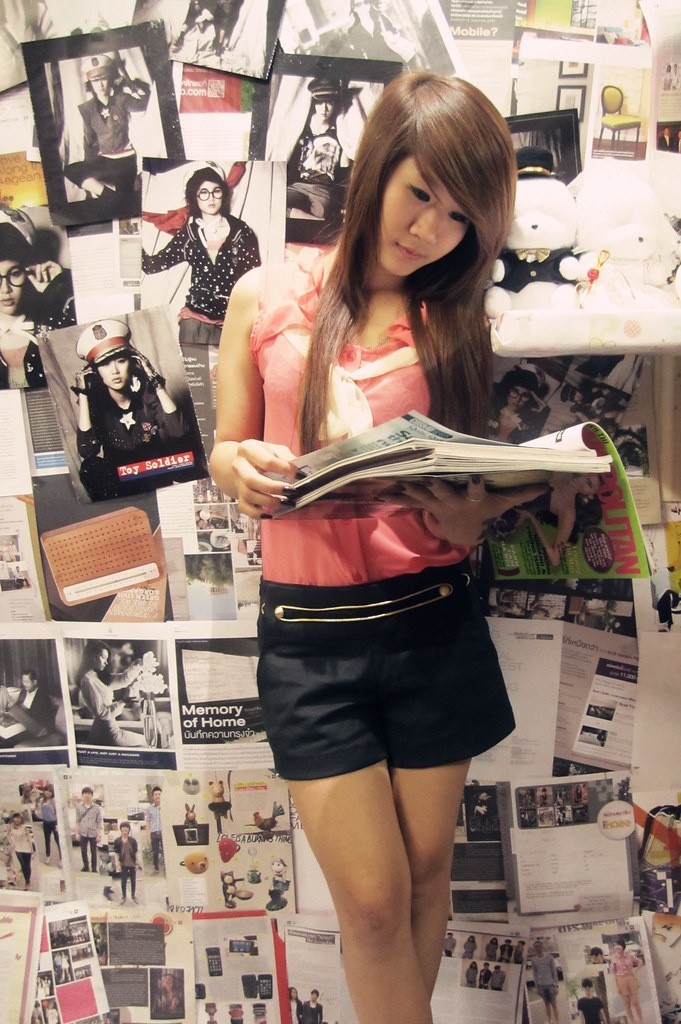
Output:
[261,409,655,580]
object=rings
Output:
[465,493,485,502]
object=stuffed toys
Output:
[484,156,681,320]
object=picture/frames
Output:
[558,61,589,77]
[556,84,586,122]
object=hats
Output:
[0,222,31,260]
[75,319,132,365]
[308,77,342,101]
[515,144,556,175]
[80,54,114,80]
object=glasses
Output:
[0,266,34,287]
[196,186,224,201]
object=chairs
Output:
[598,85,641,155]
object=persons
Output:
[488,365,538,443]
[207,69,545,1024]
[496,473,603,567]
[0,640,166,904]
[0,0,413,500]
[561,380,626,432]
[287,987,323,1024]
[444,925,645,1024]
